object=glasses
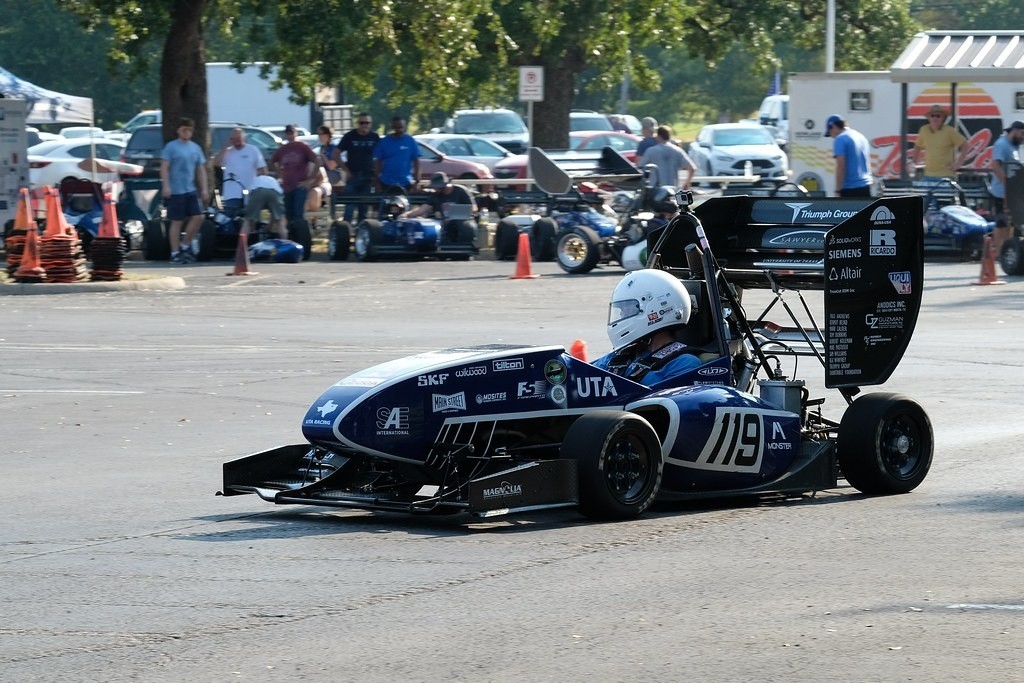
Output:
[930,113,941,118]
[357,121,371,125]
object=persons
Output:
[161,111,477,265]
[635,116,698,190]
[591,268,702,387]
[988,120,1024,262]
[824,114,873,198]
[912,105,968,209]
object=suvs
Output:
[119,124,287,191]
[756,94,789,148]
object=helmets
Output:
[607,269,691,354]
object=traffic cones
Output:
[509,233,542,280]
[2,184,128,284]
[970,237,1007,286]
[569,339,587,363]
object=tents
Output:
[0,65,96,182]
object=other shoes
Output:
[180,245,197,263]
[168,252,186,264]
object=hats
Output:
[643,116,659,132]
[285,125,296,133]
[823,114,843,137]
[176,117,195,128]
[425,171,453,189]
[1002,121,1024,131]
[924,104,948,116]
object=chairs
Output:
[674,277,717,359]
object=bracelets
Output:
[835,190,841,193]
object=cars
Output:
[267,106,683,189]
[687,123,789,190]
[25,110,162,190]
